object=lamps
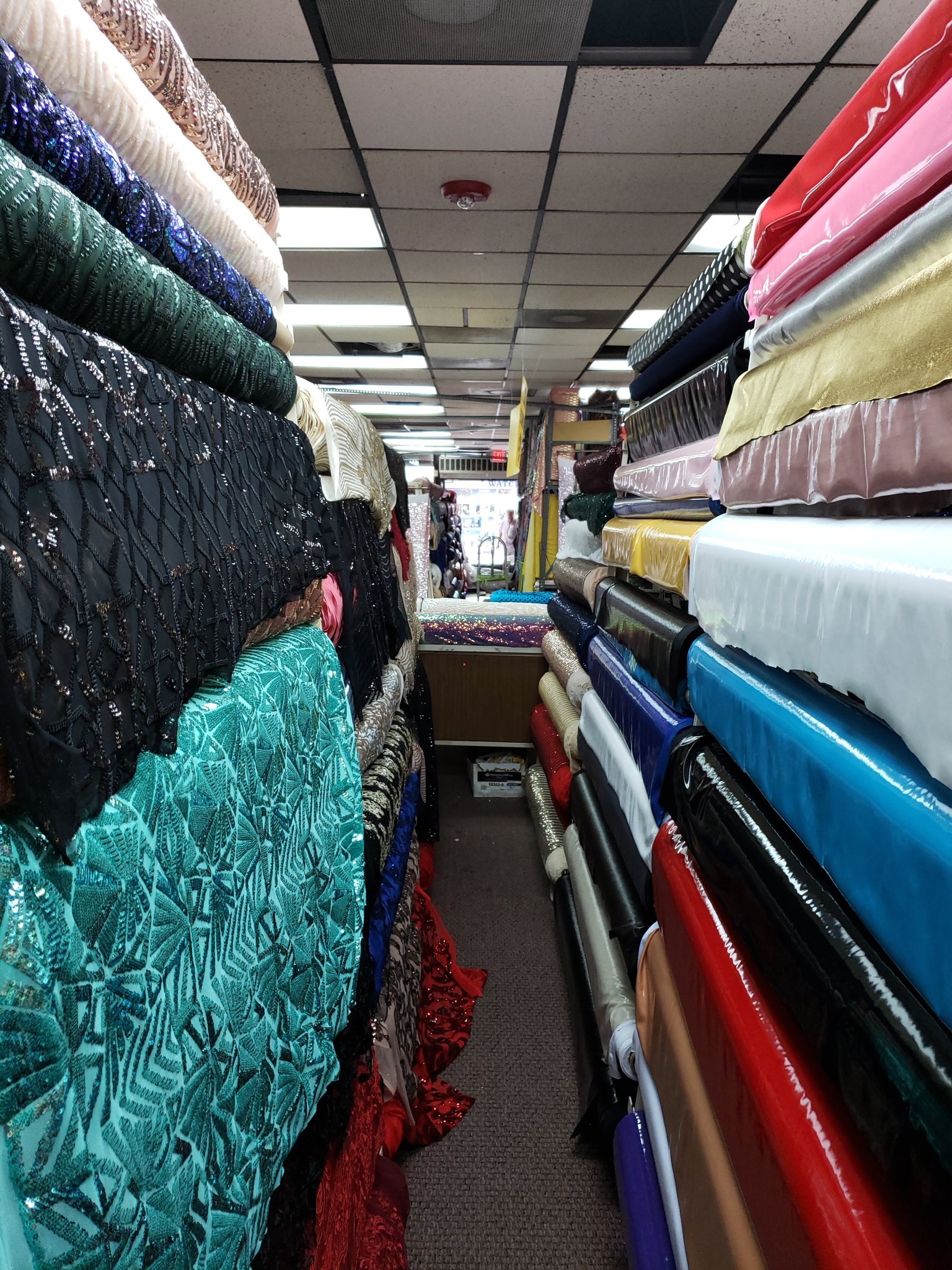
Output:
[440,179,492,212]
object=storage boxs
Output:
[465,749,531,799]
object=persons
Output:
[496,509,518,555]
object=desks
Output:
[415,640,553,750]
